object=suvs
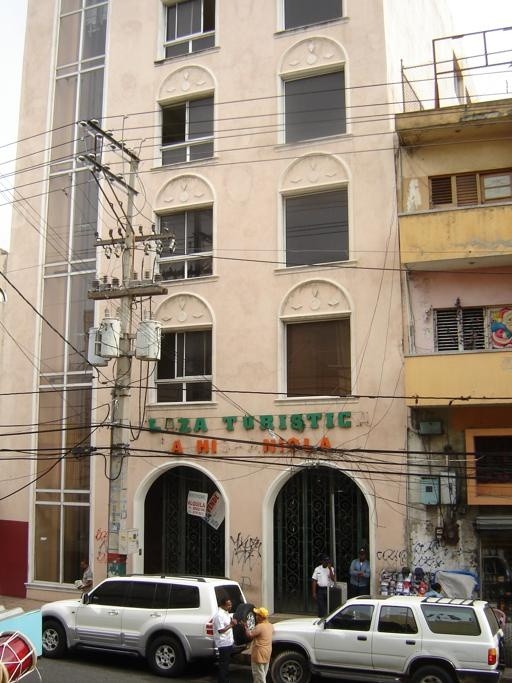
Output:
[40,574,258,677]
[270,595,506,683]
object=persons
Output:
[239,604,276,683]
[490,607,506,660]
[211,598,237,681]
[311,554,336,617]
[74,559,92,594]
[425,582,443,597]
[348,547,370,593]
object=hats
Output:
[253,608,269,618]
